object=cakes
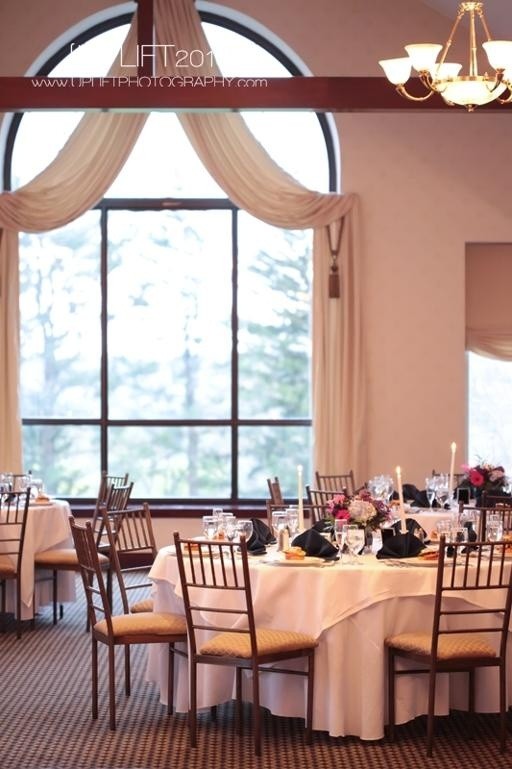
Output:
[285,546,306,560]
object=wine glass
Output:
[0,471,55,506]
[365,472,512,514]
[202,507,512,567]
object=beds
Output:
[1,496,76,621]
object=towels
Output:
[249,518,276,544]
[237,530,267,555]
[291,529,340,562]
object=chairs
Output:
[172,531,320,756]
[68,515,188,731]
[383,489,512,759]
[265,469,356,537]
[0,487,30,639]
[433,469,465,491]
[30,469,158,633]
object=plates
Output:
[167,546,220,557]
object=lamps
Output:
[376,0,511,113]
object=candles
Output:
[396,467,408,533]
[449,441,458,488]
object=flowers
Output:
[324,488,390,534]
[470,461,508,487]
